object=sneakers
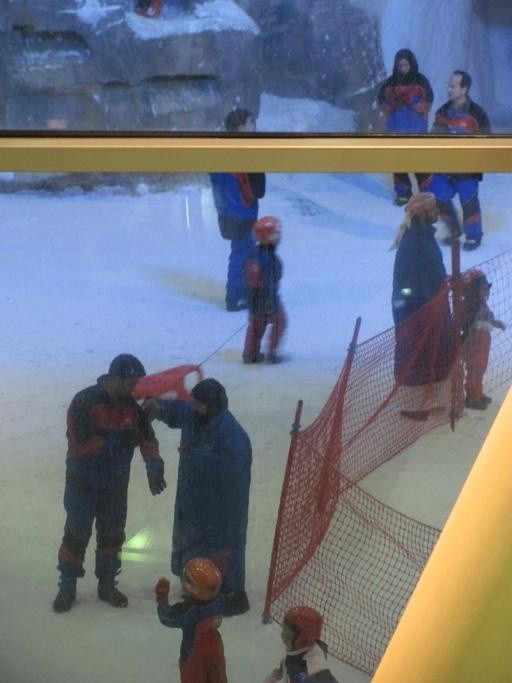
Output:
[402,410,430,421]
[98,586,128,608]
[464,394,493,410]
[239,350,284,365]
[446,230,483,248]
[225,300,250,312]
[392,193,407,207]
[52,587,77,613]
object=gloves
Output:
[215,552,229,569]
[147,470,167,496]
[153,577,170,604]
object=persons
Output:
[455,268,505,409]
[211,108,265,310]
[156,553,248,683]
[143,376,250,613]
[52,353,167,610]
[376,47,435,205]
[387,190,454,420]
[266,605,338,682]
[241,215,292,362]
[430,69,490,250]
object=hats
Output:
[191,378,229,416]
[403,192,436,215]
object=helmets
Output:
[180,558,222,601]
[253,216,282,245]
[460,268,486,287]
[283,606,324,649]
